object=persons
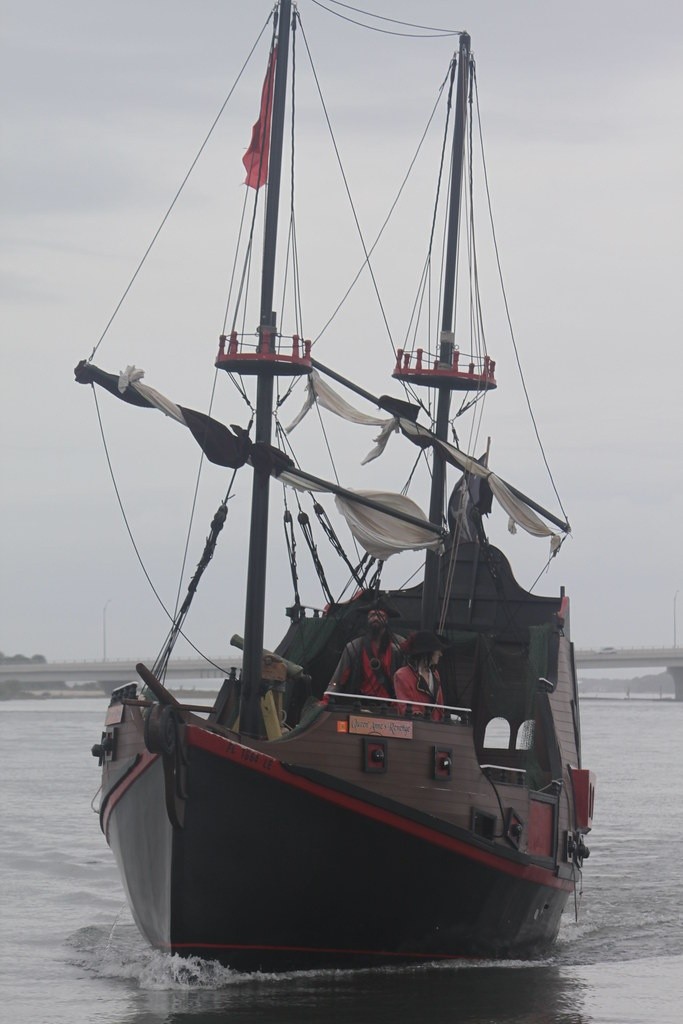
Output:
[323,594,408,714]
[393,629,452,722]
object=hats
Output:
[403,631,452,654]
[356,599,400,618]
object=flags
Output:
[242,43,278,191]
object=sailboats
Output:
[71,1,600,980]
